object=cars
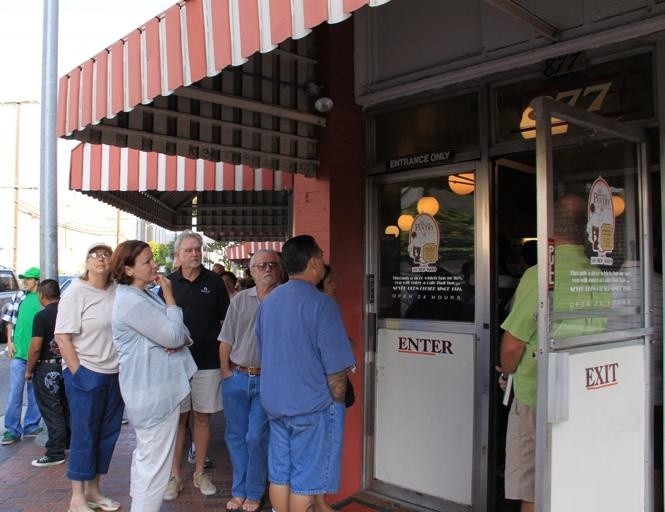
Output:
[0,271,27,343]
[57,274,82,292]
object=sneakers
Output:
[122,419,129,425]
[0,431,19,446]
[31,453,66,468]
[163,476,186,503]
[193,471,219,497]
[23,427,44,439]
[187,447,215,469]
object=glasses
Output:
[87,250,113,260]
[251,261,282,271]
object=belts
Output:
[36,359,62,365]
[228,363,261,376]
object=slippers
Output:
[225,497,244,512]
[241,499,263,512]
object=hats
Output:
[86,241,113,258]
[17,266,41,281]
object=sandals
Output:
[85,498,122,512]
[67,505,97,512]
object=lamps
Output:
[519,105,568,139]
[612,194,625,217]
[384,172,474,238]
[313,85,335,112]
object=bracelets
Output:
[500,374,507,381]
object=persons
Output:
[25,280,71,467]
[53,242,125,512]
[0,268,44,445]
[496,194,612,511]
[316,266,338,512]
[157,232,232,501]
[187,407,214,468]
[217,250,283,512]
[110,241,198,512]
[2,289,27,360]
[220,272,241,301]
[254,235,355,512]
[212,264,225,274]
